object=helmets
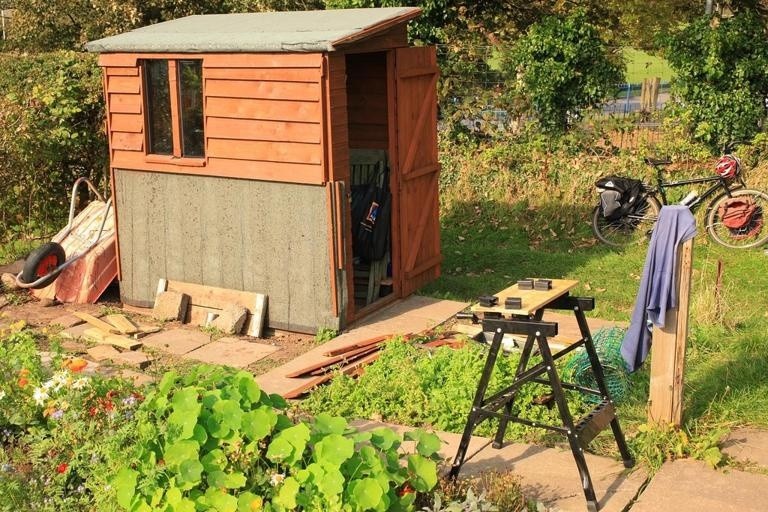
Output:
[715,155,737,180]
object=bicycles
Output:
[590,134,768,250]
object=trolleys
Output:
[17,176,118,306]
